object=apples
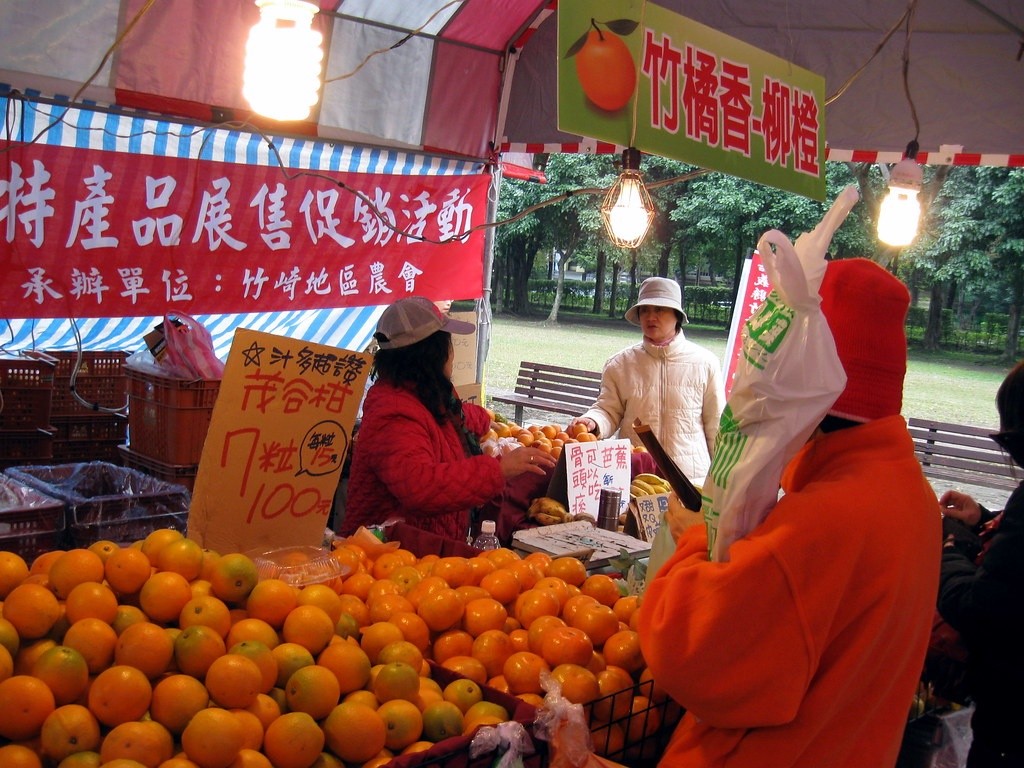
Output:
[907,686,928,720]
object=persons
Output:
[570,276,726,489]
[640,260,943,768]
[339,295,558,543]
[937,361,1024,767]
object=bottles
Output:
[473,520,501,549]
[597,488,622,532]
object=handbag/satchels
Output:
[699,184,861,566]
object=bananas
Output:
[527,496,569,526]
[617,472,703,527]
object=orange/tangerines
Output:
[0,528,677,768]
[577,29,637,111]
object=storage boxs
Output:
[0,349,226,565]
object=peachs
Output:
[476,408,599,460]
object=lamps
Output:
[601,0,656,250]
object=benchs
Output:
[908,418,1024,492]
[491,361,603,427]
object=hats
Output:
[624,277,689,327]
[376,296,475,349]
[820,258,912,421]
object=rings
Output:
[530,456,534,463]
